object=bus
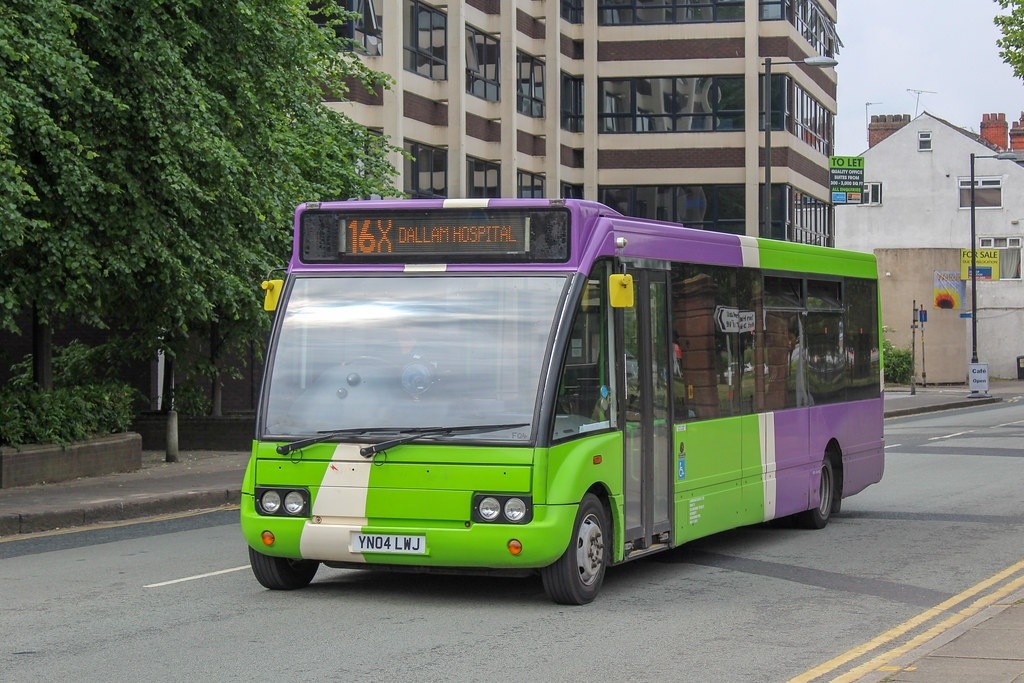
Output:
[240,197,886,606]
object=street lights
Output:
[764,56,838,240]
[970,154,1018,394]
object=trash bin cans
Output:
[1017,355,1024,379]
